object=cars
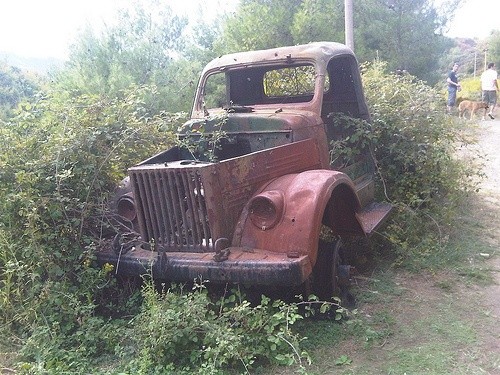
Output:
[91,43,397,300]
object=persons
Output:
[480,62,500,121]
[446,63,461,114]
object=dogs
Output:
[458,100,489,119]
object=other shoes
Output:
[488,113,494,119]
[481,115,486,121]
